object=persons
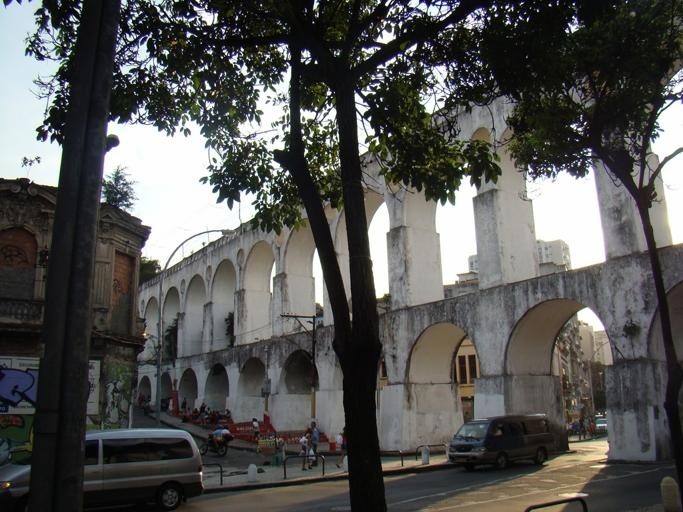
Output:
[222,424,230,441]
[106,380,117,415]
[310,421,320,467]
[335,426,348,468]
[181,397,187,413]
[588,416,593,423]
[184,402,231,425]
[109,401,119,424]
[211,424,221,448]
[252,418,261,444]
[589,421,596,437]
[299,431,312,471]
[493,426,503,436]
[578,415,586,440]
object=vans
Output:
[0,427,205,512]
[447,413,555,472]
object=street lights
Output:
[155,228,236,428]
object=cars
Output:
[595,418,608,433]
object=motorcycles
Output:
[199,432,234,457]
